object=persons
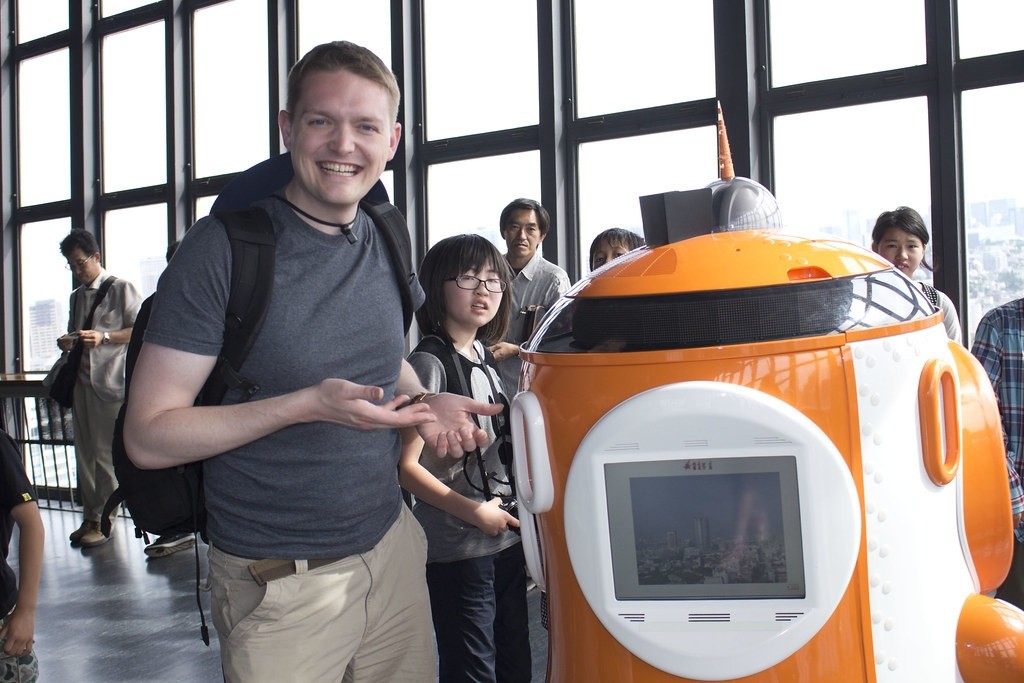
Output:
[0,427,46,683]
[55,229,145,547]
[869,207,963,348]
[477,197,572,597]
[397,234,535,683]
[124,41,505,683]
[590,227,646,272]
[971,296,1023,611]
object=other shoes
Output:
[69,519,94,543]
[144,532,196,558]
[80,521,115,547]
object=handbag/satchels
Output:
[41,349,82,407]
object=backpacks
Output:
[102,198,413,543]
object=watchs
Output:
[409,393,436,406]
[102,332,111,344]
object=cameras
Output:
[499,497,521,536]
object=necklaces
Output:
[270,188,360,245]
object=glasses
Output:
[65,254,94,271]
[443,275,508,293]
